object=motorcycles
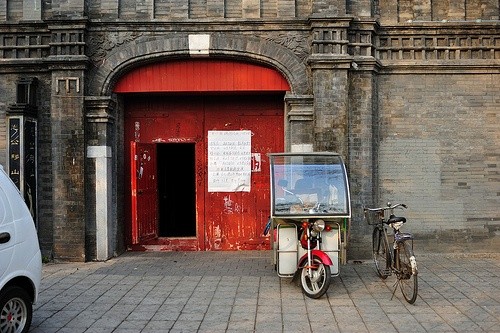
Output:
[263,151,353,298]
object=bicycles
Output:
[362,202,421,305]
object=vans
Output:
[0,162,44,333]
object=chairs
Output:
[285,178,323,202]
